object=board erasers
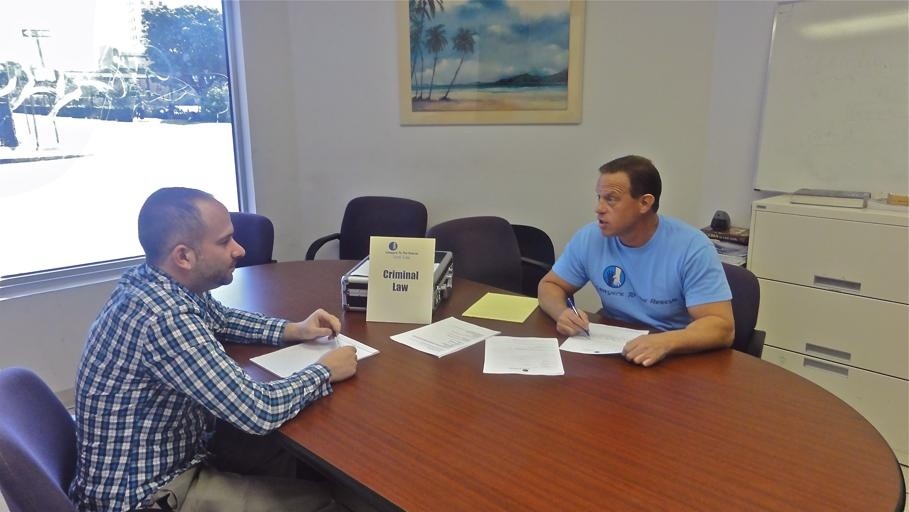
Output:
[887,193,908,207]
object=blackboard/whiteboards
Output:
[752,0,908,195]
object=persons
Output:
[66,187,359,512]
[537,156,735,368]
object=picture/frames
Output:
[396,0,586,125]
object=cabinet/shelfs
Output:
[748,192,909,512]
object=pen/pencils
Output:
[567,297,590,337]
[332,330,340,348]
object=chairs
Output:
[304,196,428,260]
[510,225,555,299]
[721,262,766,360]
[229,212,278,267]
[0,369,80,512]
[426,217,523,293]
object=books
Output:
[711,239,747,266]
[790,188,871,209]
[700,224,750,246]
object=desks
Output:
[211,260,906,512]
